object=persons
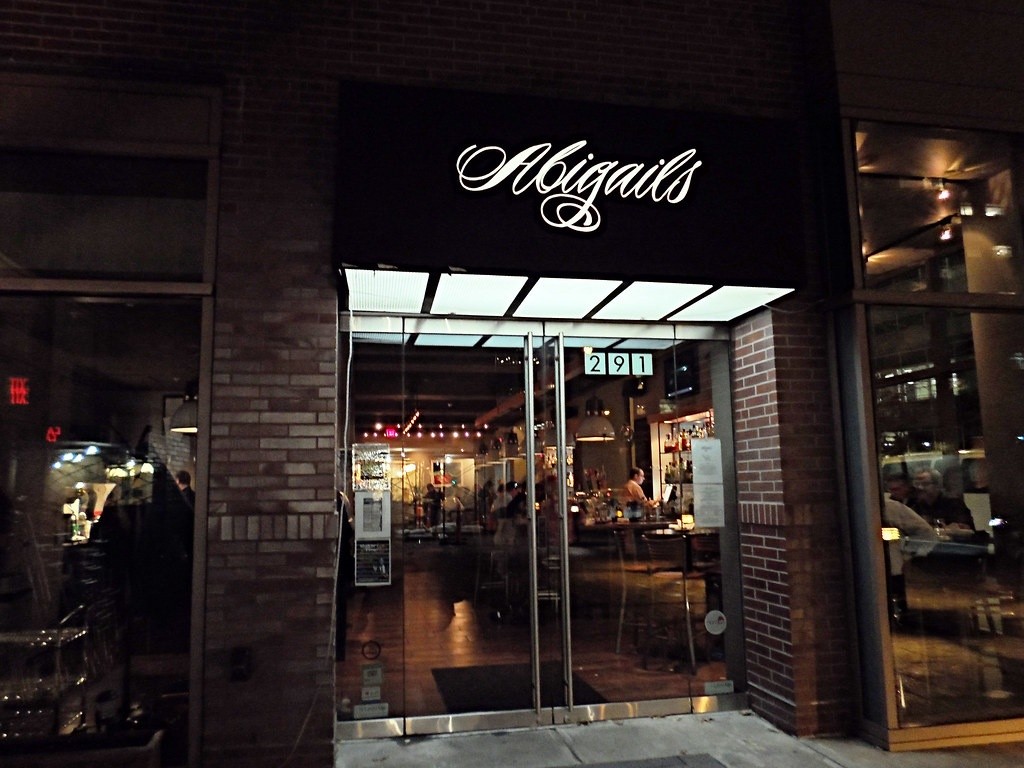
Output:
[476,474,574,606]
[622,467,659,508]
[173,471,196,507]
[81,488,96,520]
[423,483,443,528]
[882,460,994,577]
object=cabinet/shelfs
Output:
[659,450,694,485]
[0,605,93,739]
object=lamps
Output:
[475,425,544,467]
[576,379,615,442]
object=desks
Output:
[644,530,720,568]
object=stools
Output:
[615,528,724,668]
[472,543,510,610]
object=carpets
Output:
[430,659,606,714]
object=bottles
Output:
[665,457,693,484]
[664,423,711,452]
